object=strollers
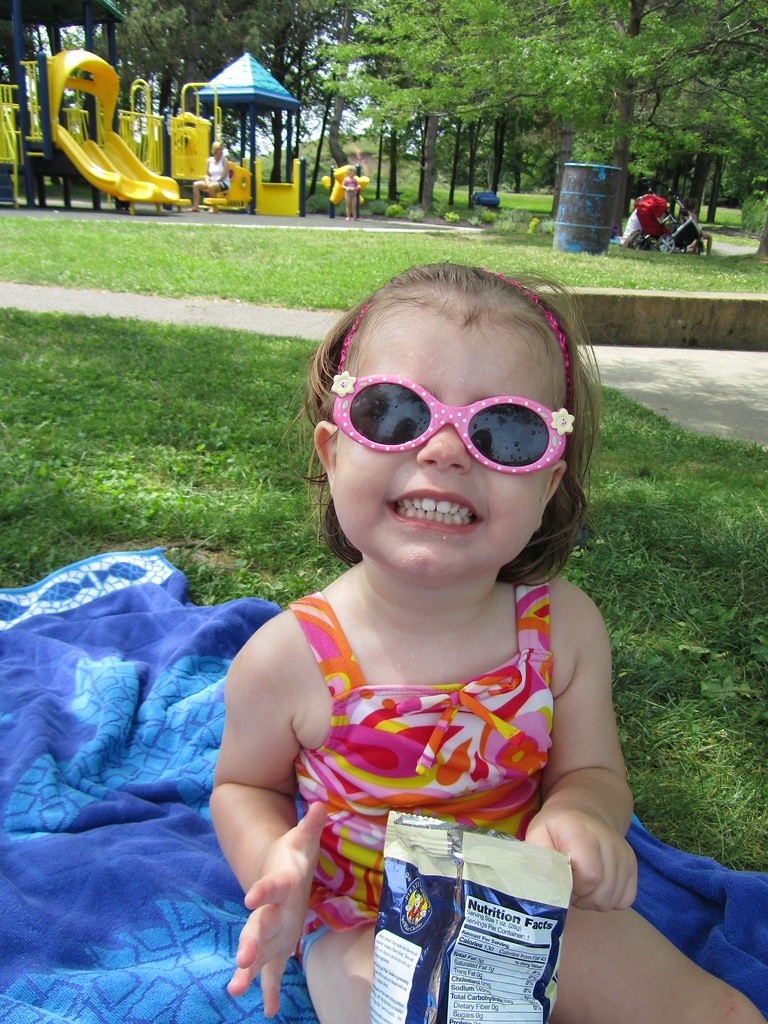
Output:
[623,177,674,252]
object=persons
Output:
[208,261,768,1024]
[681,198,713,255]
[622,208,642,238]
[341,167,361,221]
[188,141,231,214]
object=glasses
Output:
[331,371,576,476]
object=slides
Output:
[57,124,181,204]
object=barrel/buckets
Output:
[552,161,623,257]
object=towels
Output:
[0,544,767,1024]
